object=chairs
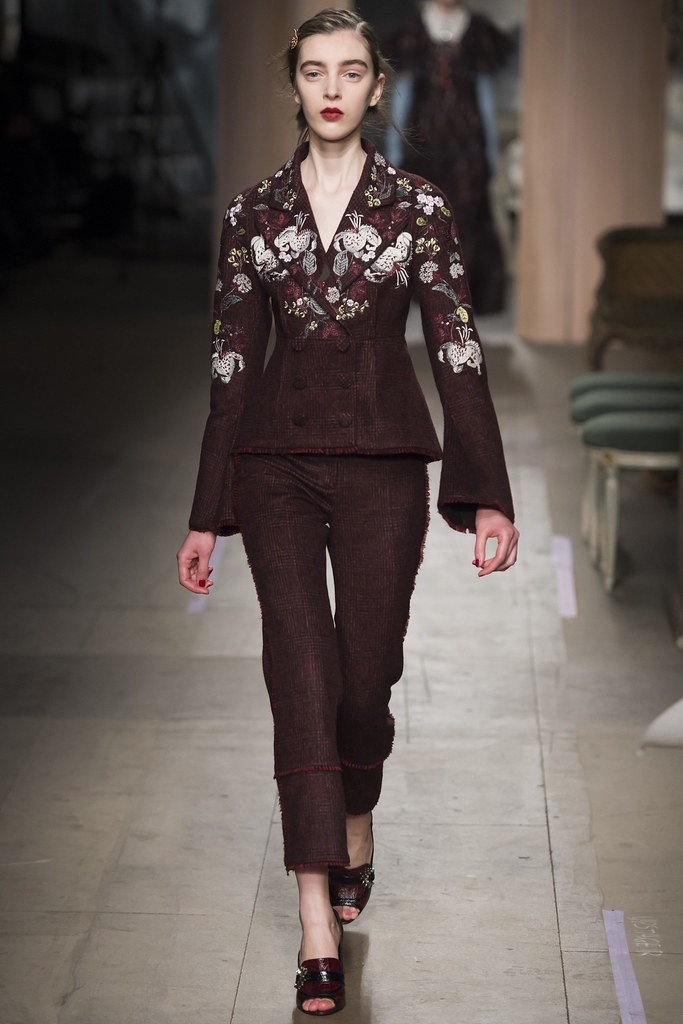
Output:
[567,368,683,596]
[585,224,683,373]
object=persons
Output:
[175,8,520,1017]
[381,0,508,314]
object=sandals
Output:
[293,908,346,1016]
[328,810,375,925]
